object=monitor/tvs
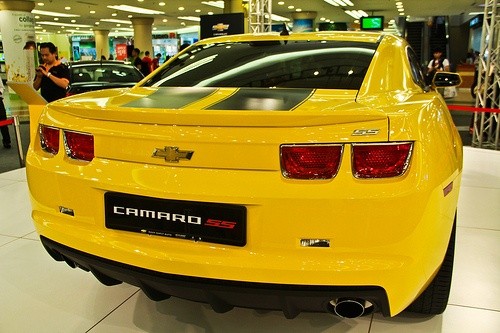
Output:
[0,51,5,64]
[360,16,383,30]
[200,13,244,40]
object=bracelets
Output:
[46,72,51,77]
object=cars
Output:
[22,21,465,321]
[65,49,148,98]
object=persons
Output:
[33,42,72,103]
[132,47,161,75]
[427,47,450,72]
[0,78,13,149]
